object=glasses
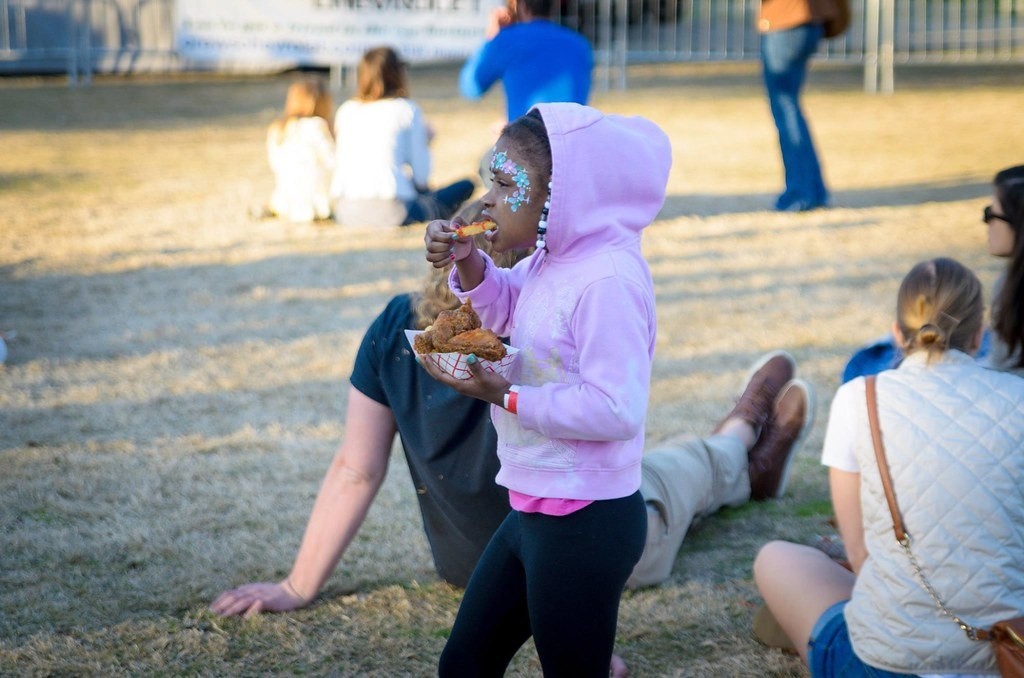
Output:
[982,205,1013,224]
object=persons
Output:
[756,0,829,211]
[421,102,673,678]
[333,48,474,228]
[266,75,336,221]
[207,196,814,621]
[753,166,1024,678]
[460,0,597,126]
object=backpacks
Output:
[807,0,851,38]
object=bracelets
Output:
[503,384,522,415]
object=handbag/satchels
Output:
[983,616,1024,678]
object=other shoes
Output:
[753,604,799,655]
[747,378,817,500]
[709,349,796,460]
